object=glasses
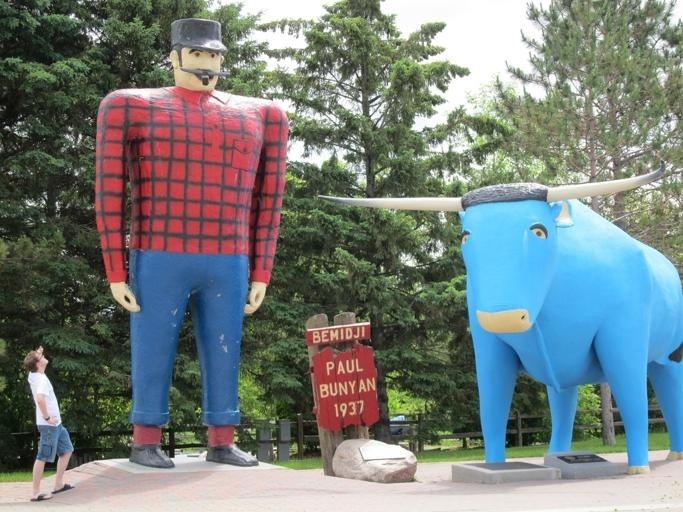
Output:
[38,355,45,360]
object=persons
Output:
[22,345,75,502]
[92,17,289,467]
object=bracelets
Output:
[42,415,51,422]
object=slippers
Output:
[30,493,53,501]
[51,483,75,494]
